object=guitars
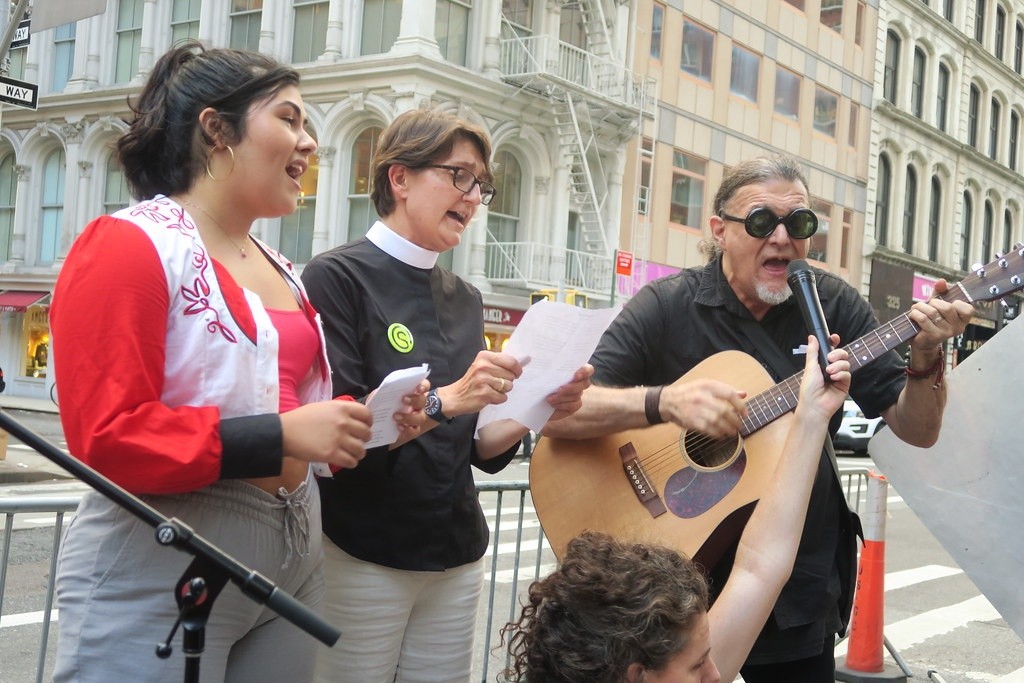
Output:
[529,239,1023,571]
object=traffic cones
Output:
[828,471,907,683]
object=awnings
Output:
[0,290,51,313]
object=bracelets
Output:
[904,345,944,390]
[644,386,668,425]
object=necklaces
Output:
[177,196,249,258]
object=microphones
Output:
[786,259,839,384]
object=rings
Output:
[498,378,504,392]
[932,317,945,324]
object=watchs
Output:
[424,387,455,425]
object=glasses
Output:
[724,206,818,239]
[406,163,497,206]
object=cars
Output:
[833,393,887,458]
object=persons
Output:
[492,333,850,683]
[540,155,974,683]
[300,110,594,683]
[50,39,431,683]
